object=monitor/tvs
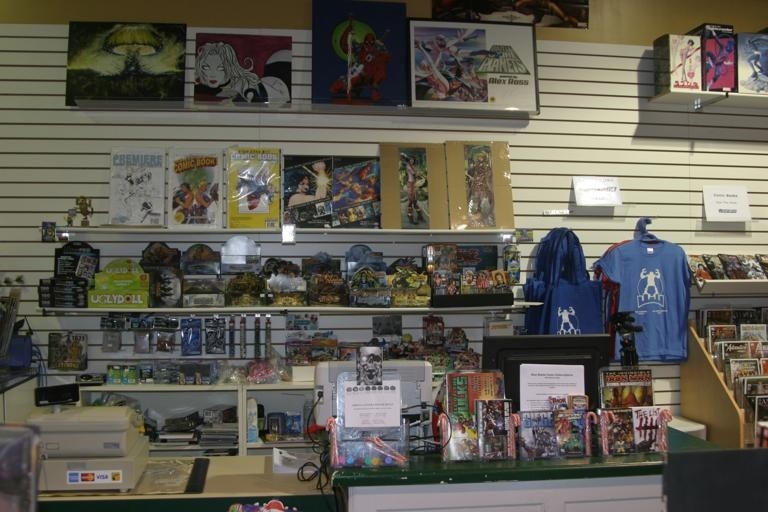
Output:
[482,334,611,414]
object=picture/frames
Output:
[409,16,540,115]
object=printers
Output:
[315,359,432,428]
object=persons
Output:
[514,0,580,29]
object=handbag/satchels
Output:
[523,227,606,336]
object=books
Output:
[682,250,766,449]
[436,365,668,463]
[106,137,515,233]
[145,404,239,459]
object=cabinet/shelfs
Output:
[680,327,767,450]
[35,226,543,454]
